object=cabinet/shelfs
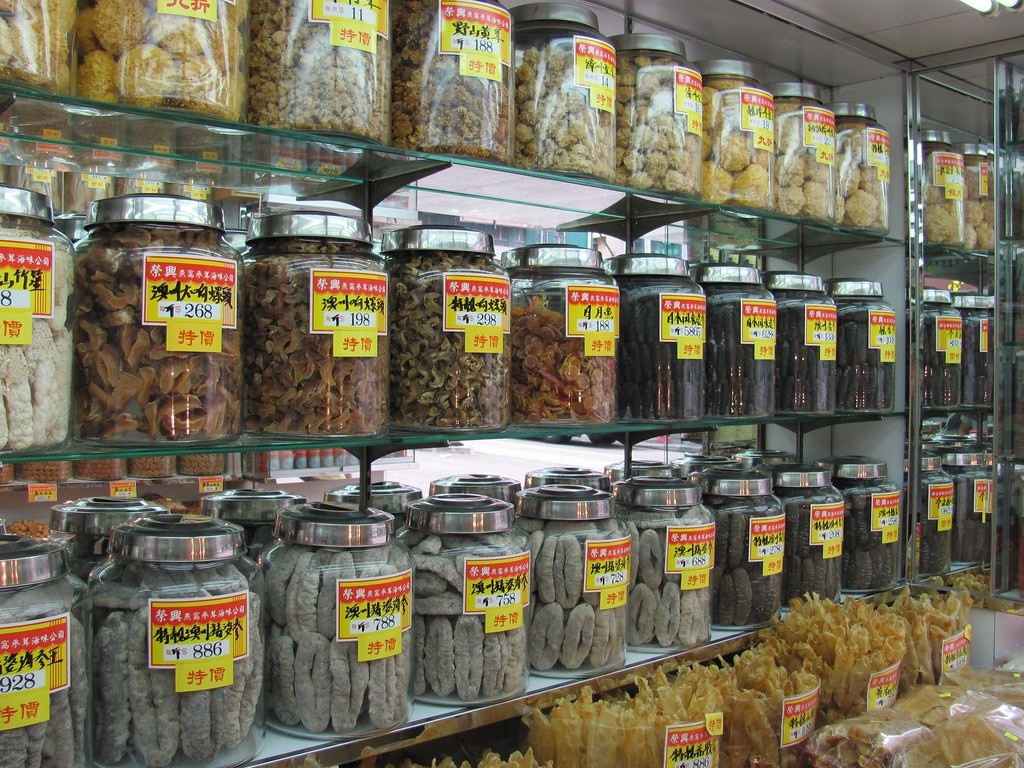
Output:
[0,0,909,768]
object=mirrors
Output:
[910,35,1024,616]
